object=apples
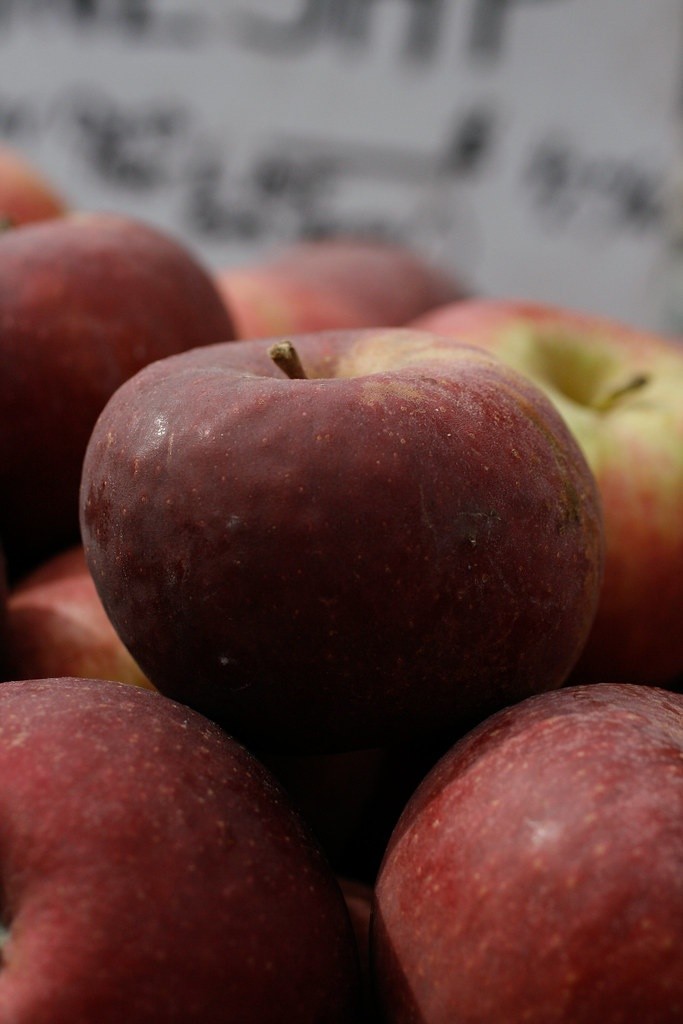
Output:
[0,148,683,1024]
